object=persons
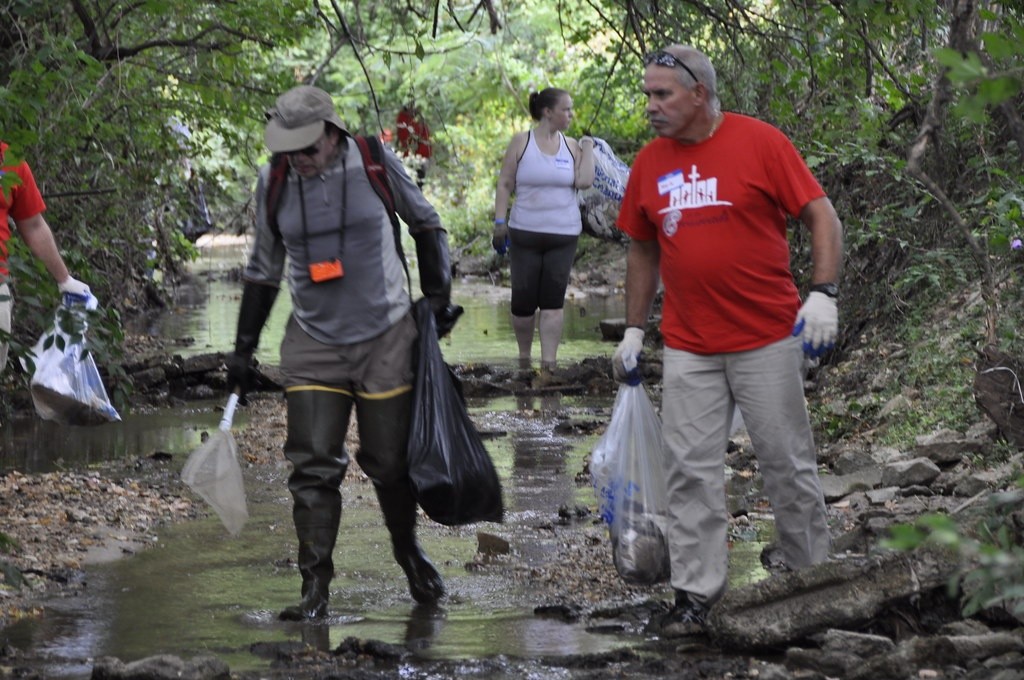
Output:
[0,138,98,381]
[227,85,452,622]
[610,45,845,629]
[494,87,595,384]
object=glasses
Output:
[643,50,698,83]
[283,145,318,157]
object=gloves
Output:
[224,356,250,407]
[55,274,99,311]
[492,219,511,255]
[610,326,646,387]
[790,290,841,361]
[578,135,594,149]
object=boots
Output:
[278,385,355,621]
[349,384,445,605]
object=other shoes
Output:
[660,579,728,639]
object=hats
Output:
[263,84,352,154]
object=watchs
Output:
[809,281,842,297]
[578,138,594,150]
